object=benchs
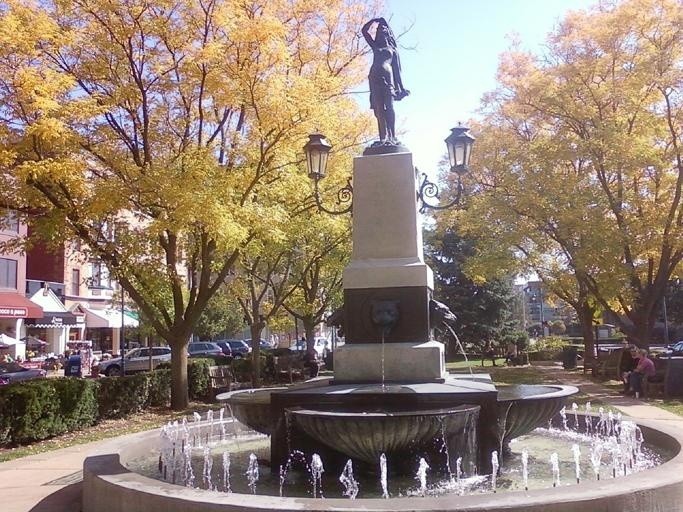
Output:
[207,353,313,400]
[480,345,510,366]
[639,356,671,402]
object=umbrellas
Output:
[21,336,47,345]
[0,333,25,347]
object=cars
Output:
[0,361,46,385]
[655,340,683,358]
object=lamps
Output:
[303,132,352,217]
[414,121,476,215]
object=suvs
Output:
[289,337,345,359]
[188,339,270,357]
[96,346,190,377]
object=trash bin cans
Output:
[562,345,578,370]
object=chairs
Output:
[582,347,625,378]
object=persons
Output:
[503,339,520,362]
[361,17,409,144]
[27,350,35,358]
[541,322,549,337]
[2,354,8,361]
[482,339,499,366]
[620,346,642,395]
[8,353,13,362]
[532,328,538,340]
[630,349,657,399]
